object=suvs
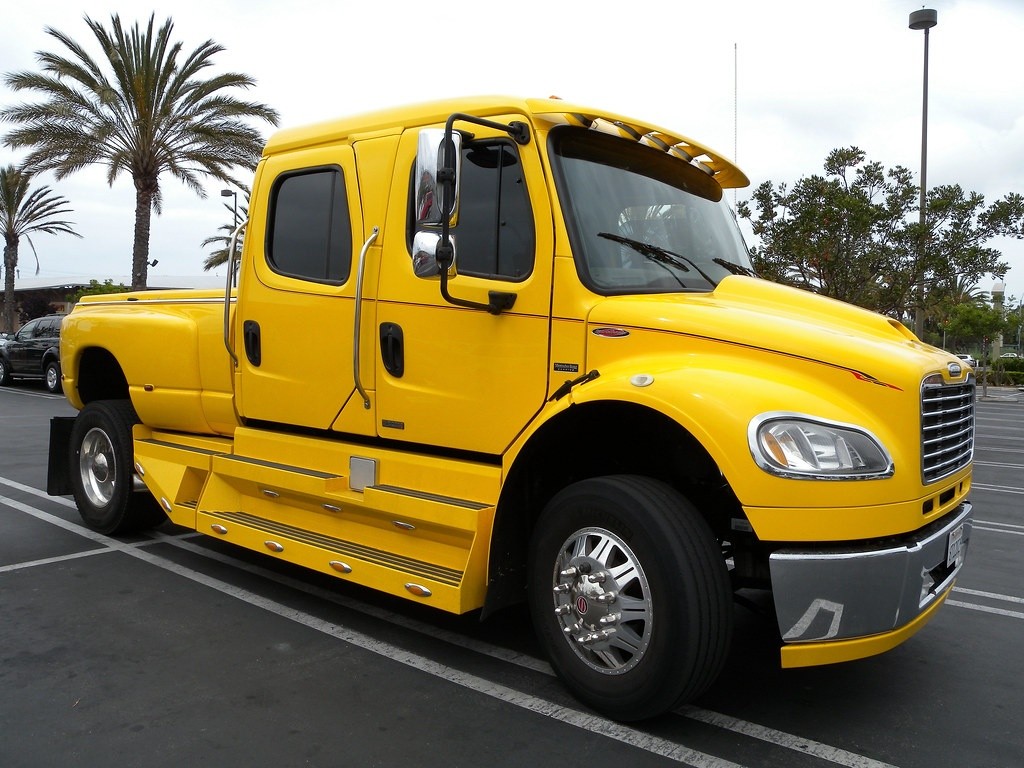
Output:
[0,312,71,394]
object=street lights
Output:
[219,189,237,289]
[907,5,939,343]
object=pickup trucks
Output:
[45,93,978,727]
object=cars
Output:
[954,354,976,368]
[1000,352,1023,358]
